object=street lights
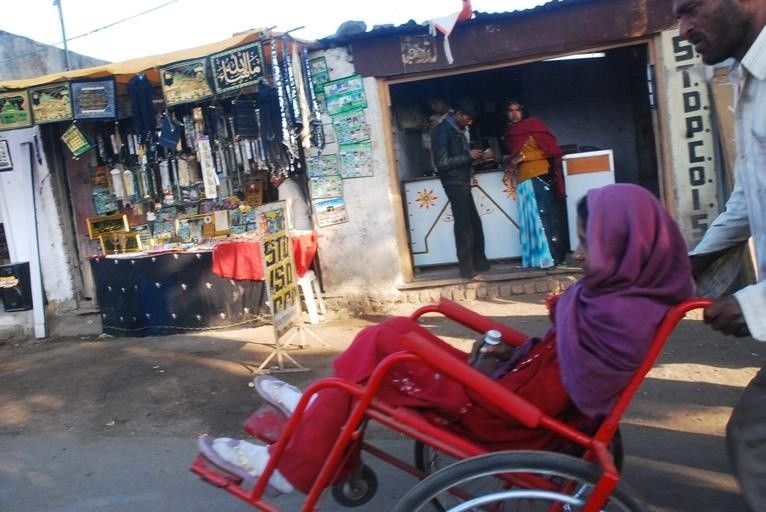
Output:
[54,0,71,70]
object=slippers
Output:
[197,433,282,498]
[254,373,304,420]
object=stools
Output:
[297,270,326,325]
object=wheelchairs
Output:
[188,296,718,512]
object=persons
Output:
[501,96,570,269]
[430,96,491,281]
[428,91,485,162]
[197,182,699,498]
[672,1,766,511]
[270,171,311,231]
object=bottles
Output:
[472,328,502,378]
